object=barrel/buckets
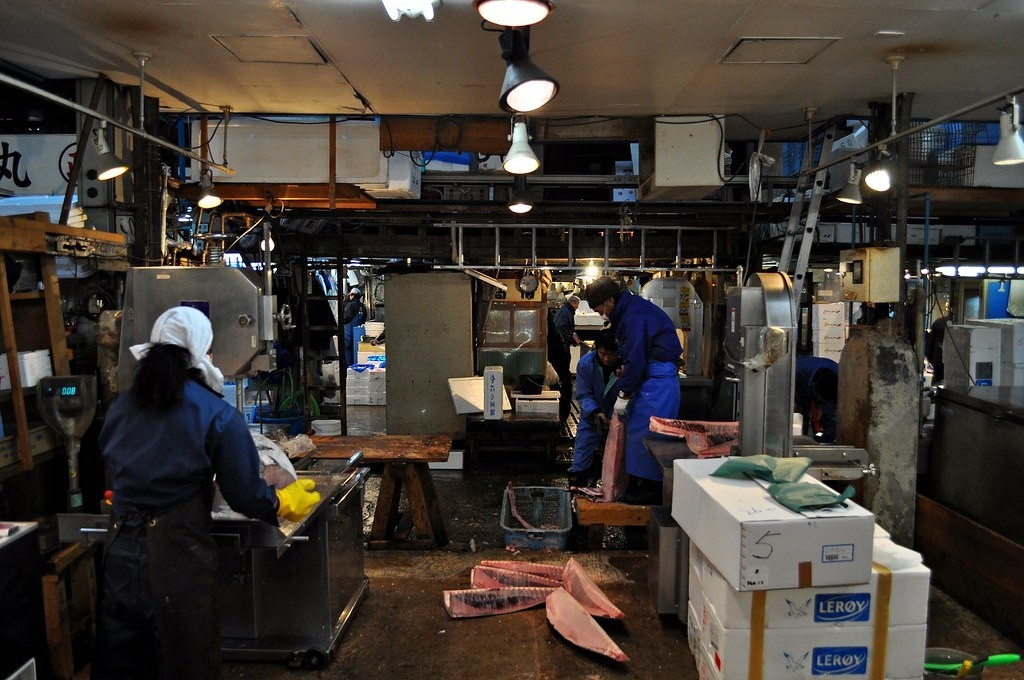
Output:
[924,647,985,680]
[310,419,341,435]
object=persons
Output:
[370,324,385,347]
[584,276,685,503]
[794,357,839,443]
[547,295,581,384]
[567,329,624,482]
[343,287,367,367]
[90,306,321,680]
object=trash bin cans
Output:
[922,647,985,680]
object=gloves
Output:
[614,396,631,419]
[276,479,321,523]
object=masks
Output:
[600,314,610,322]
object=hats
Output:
[587,276,620,310]
[348,288,361,296]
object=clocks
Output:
[519,275,539,292]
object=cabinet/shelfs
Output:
[0,215,128,473]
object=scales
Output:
[35,372,100,550]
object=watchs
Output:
[618,390,633,397]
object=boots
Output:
[567,470,589,494]
[621,474,663,505]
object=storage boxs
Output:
[427,450,466,469]
[346,321,386,405]
[937,144,1024,188]
[500,485,573,550]
[0,350,54,391]
[0,427,54,468]
[941,316,1024,388]
[516,397,559,415]
[356,145,420,199]
[811,301,846,363]
[671,458,930,680]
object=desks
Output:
[299,435,454,549]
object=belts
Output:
[120,526,147,536]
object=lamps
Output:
[198,164,223,208]
[380,0,441,22]
[92,119,130,180]
[473,0,562,213]
[865,144,894,192]
[835,157,863,204]
[993,92,1024,165]
